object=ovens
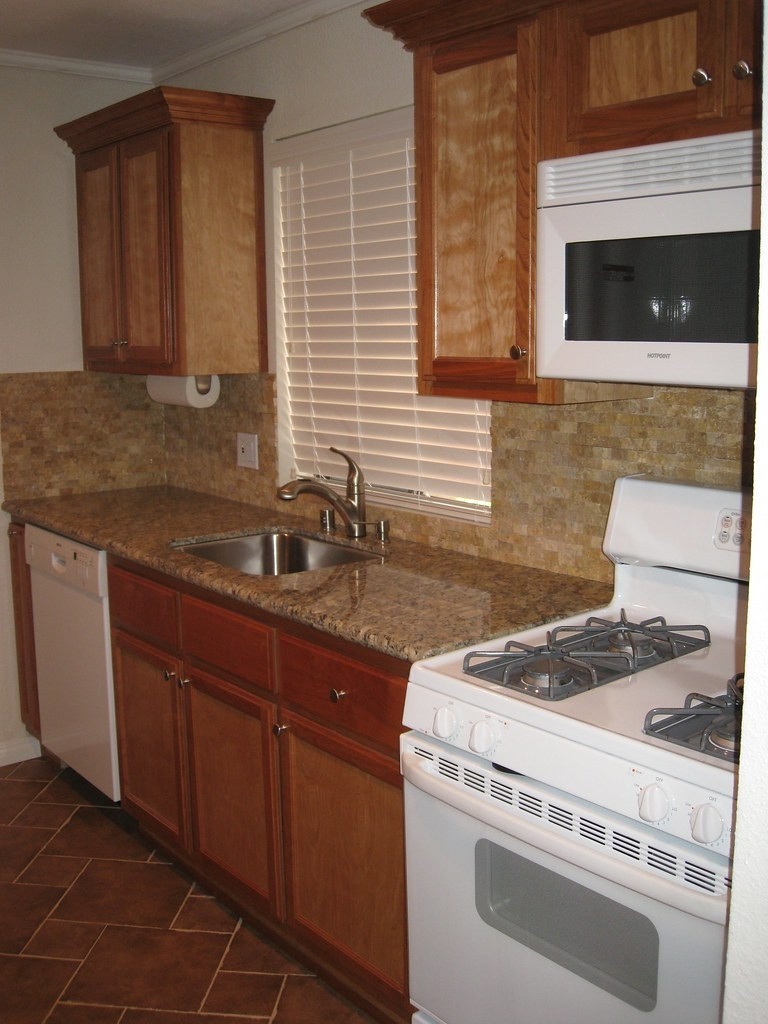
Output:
[401,731,767,1023]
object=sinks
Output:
[173,523,388,580]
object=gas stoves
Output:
[400,471,755,863]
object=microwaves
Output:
[533,125,761,391]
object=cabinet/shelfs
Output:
[108,568,283,950]
[361,0,564,407]
[275,633,412,1024]
[54,86,274,376]
[543,1,761,160]
[7,523,119,799]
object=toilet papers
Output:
[144,373,223,410]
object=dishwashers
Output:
[24,523,123,802]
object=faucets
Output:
[275,443,371,539]
